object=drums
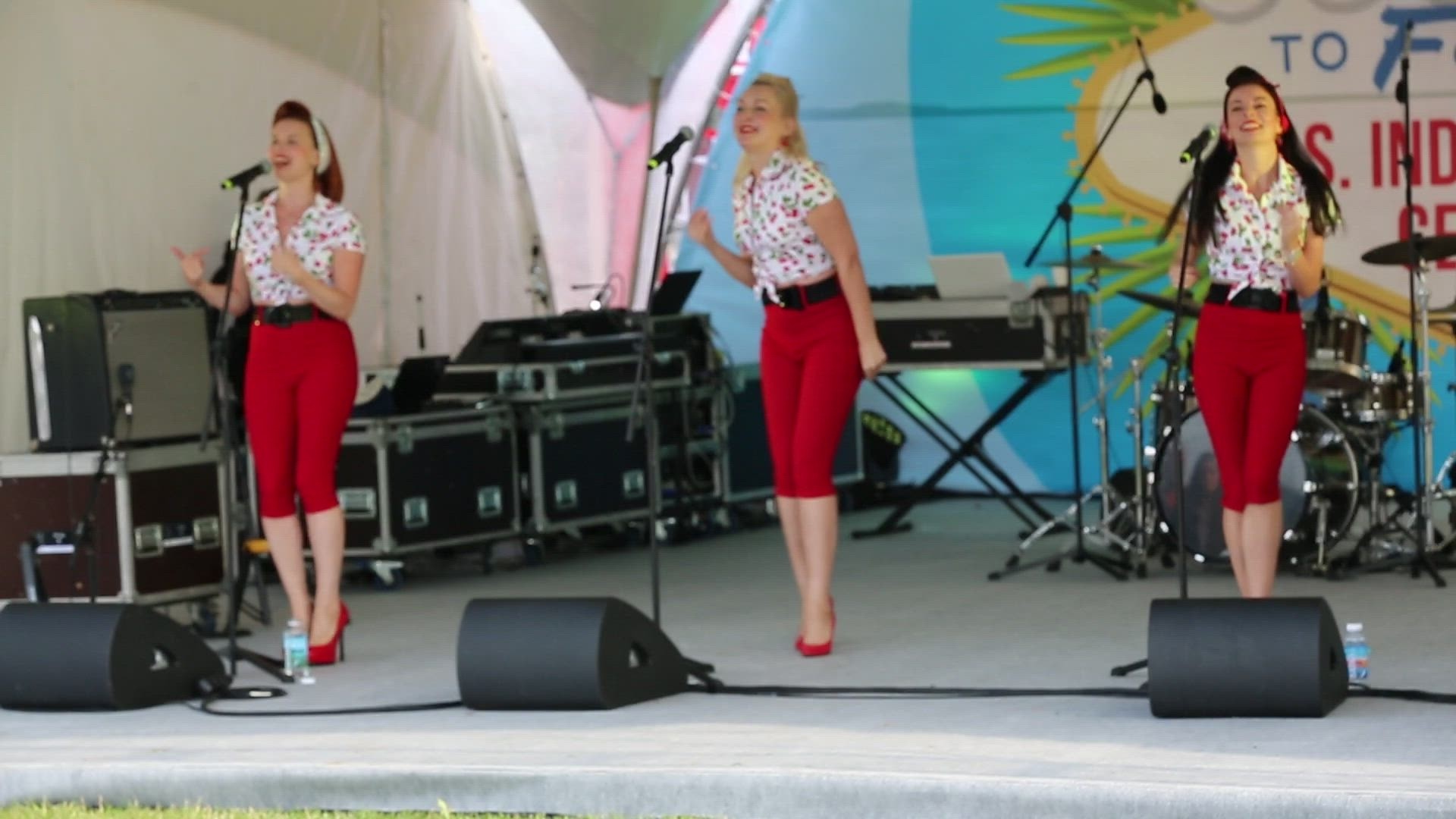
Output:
[1324,373,1414,424]
[1302,310,1369,397]
[1151,400,1358,565]
[1184,342,1196,398]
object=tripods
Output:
[985,79,1179,579]
[1321,62,1456,585]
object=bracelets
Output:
[1280,244,1303,265]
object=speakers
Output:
[0,601,226,715]
[1147,595,1349,718]
[22,290,220,451]
[457,593,689,712]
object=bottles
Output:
[1343,622,1371,688]
[281,619,310,677]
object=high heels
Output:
[795,596,835,657]
[308,599,351,666]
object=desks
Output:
[852,354,1090,539]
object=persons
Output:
[1167,65,1341,599]
[687,74,888,656]
[171,100,366,666]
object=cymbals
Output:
[1041,251,1155,270]
[1116,287,1205,319]
[1410,297,1456,336]
[1361,233,1456,266]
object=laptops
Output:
[928,253,1016,299]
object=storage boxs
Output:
[0,440,242,610]
[307,353,868,556]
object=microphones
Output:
[1180,124,1217,163]
[647,127,694,170]
[117,361,133,416]
[1396,19,1414,102]
[1137,39,1167,114]
[222,159,274,188]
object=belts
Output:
[252,302,331,325]
[1203,283,1301,313]
[760,274,837,309]
